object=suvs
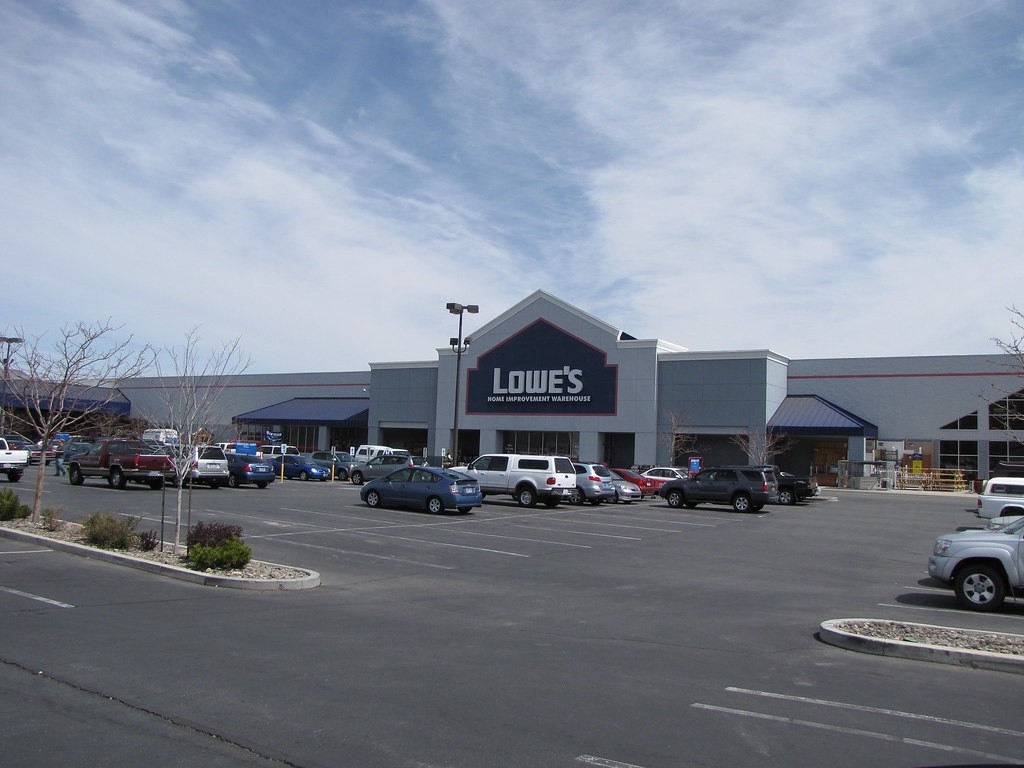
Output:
[258,445,301,465]
[311,450,360,482]
[164,443,230,490]
[977,476,1024,519]
[566,461,616,506]
[349,454,431,485]
[214,442,261,456]
[658,466,780,514]
[67,437,172,490]
[0,435,31,482]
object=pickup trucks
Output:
[750,465,819,506]
[447,453,576,508]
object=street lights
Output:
[0,335,23,436]
[445,301,479,466]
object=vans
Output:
[354,444,393,463]
[366,448,413,467]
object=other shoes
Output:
[55,474,60,476]
[63,471,66,476]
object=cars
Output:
[222,454,276,488]
[927,516,1024,613]
[608,469,642,503]
[428,456,452,468]
[55,442,95,465]
[268,455,332,482]
[609,469,656,500]
[36,438,65,455]
[641,467,692,493]
[358,466,483,515]
[25,444,56,466]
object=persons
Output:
[53,441,66,476]
